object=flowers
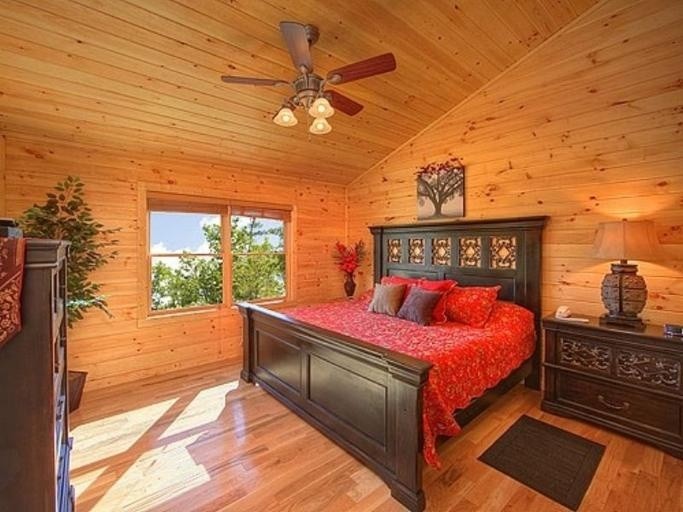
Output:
[331,239,366,272]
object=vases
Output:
[343,272,355,297]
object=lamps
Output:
[591,219,664,328]
[272,90,335,144]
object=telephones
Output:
[555,306,590,323]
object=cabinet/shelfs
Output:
[0,239,74,512]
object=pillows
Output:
[368,276,502,329]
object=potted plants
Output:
[18,176,124,412]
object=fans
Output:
[220,21,396,121]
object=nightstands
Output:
[540,312,682,461]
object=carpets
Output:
[475,414,606,512]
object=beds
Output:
[235,216,550,512]
